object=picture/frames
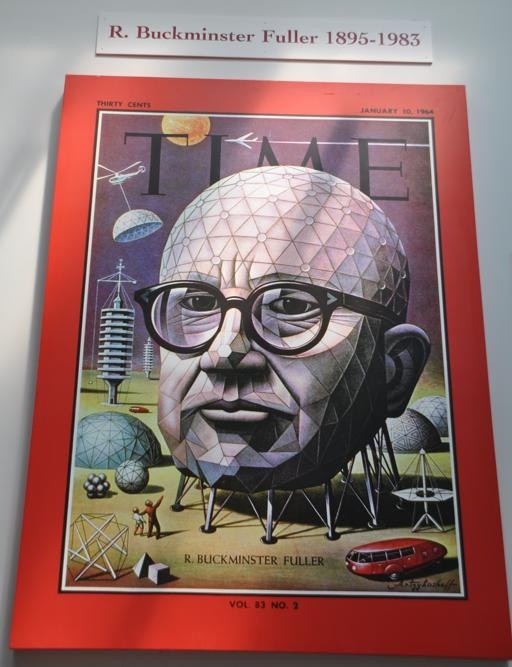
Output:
[6,70,512,664]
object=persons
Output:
[139,496,163,540]
[132,164,431,494]
[132,507,146,536]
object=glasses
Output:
[132,280,400,358]
